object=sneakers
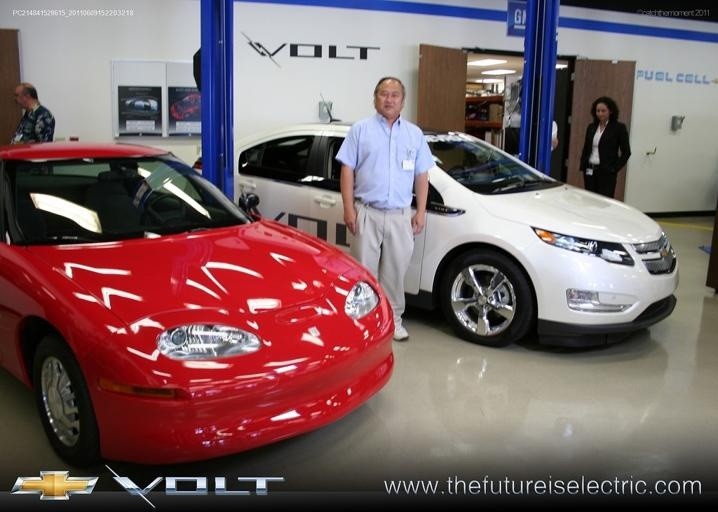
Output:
[394,325,409,341]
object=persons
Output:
[580,96,633,198]
[7,82,56,144]
[335,76,437,341]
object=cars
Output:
[192,122,680,352]
[0,141,396,467]
[168,94,202,121]
